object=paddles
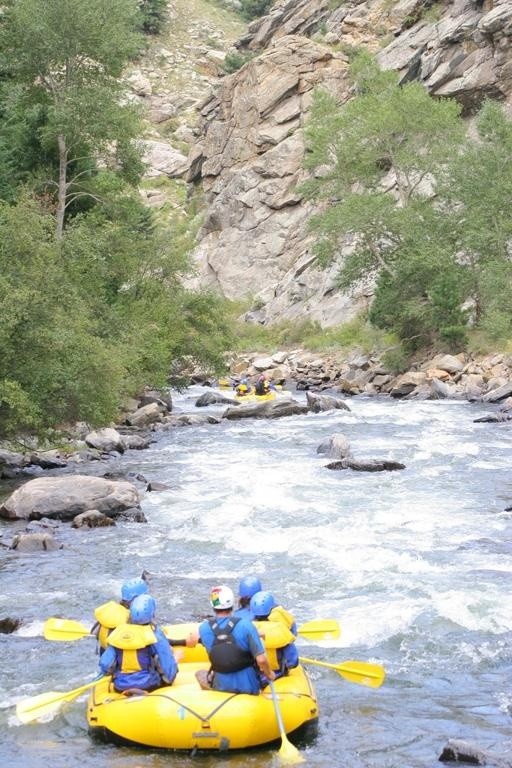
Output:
[17,675,116,725]
[44,618,97,640]
[269,680,306,766]
[299,656,385,688]
[298,621,341,641]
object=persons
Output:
[89,572,303,695]
[240,374,271,395]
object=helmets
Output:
[249,591,274,616]
[238,576,262,598]
[209,585,234,610]
[121,577,149,601]
[129,594,156,625]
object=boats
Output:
[87,622,319,751]
[219,379,282,401]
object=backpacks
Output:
[206,617,262,688]
